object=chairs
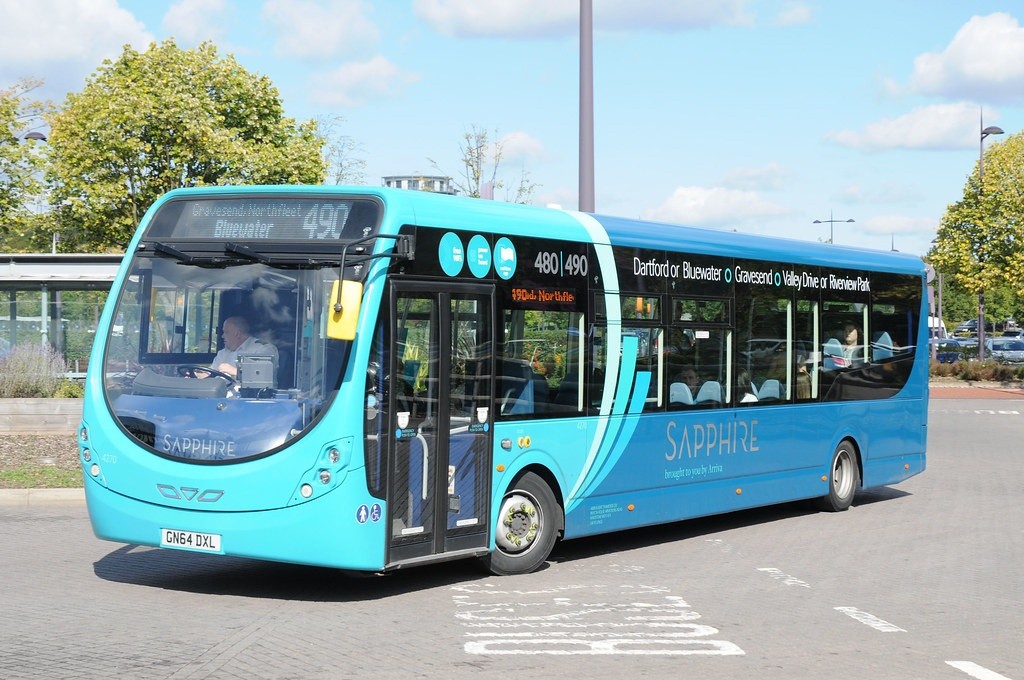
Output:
[533,329,894,414]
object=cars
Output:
[951,335,980,359]
[927,338,966,365]
[950,316,1004,339]
[986,336,1024,368]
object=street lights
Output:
[979,105,1005,362]
[812,211,855,245]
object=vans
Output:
[928,315,948,359]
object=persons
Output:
[841,323,863,368]
[185,317,279,398]
[737,367,758,402]
[396,357,414,415]
[681,365,700,397]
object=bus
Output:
[79,183,932,573]
